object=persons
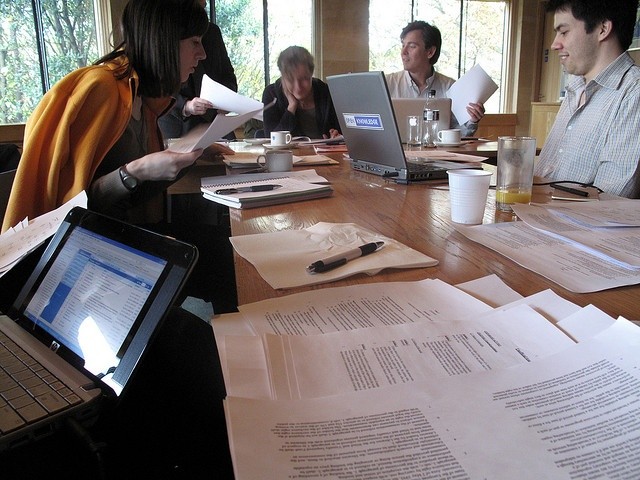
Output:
[533,0,640,199]
[1,0,207,236]
[380,20,485,139]
[262,46,347,142]
[153,0,238,141]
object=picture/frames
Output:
[626,8,639,52]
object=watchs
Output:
[122,175,139,190]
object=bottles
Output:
[421,89,440,149]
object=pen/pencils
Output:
[551,183,589,196]
[305,242,385,275]
[325,141,346,145]
[214,184,282,196]
[461,137,479,141]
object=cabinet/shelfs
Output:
[530,102,561,149]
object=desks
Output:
[227,127,639,479]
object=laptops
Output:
[326,72,483,185]
[388,97,452,143]
[1,206,197,444]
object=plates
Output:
[261,143,295,150]
[433,140,470,148]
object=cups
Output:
[494,133,538,214]
[444,166,495,227]
[438,128,462,144]
[257,150,294,174]
[269,129,293,146]
[406,113,424,150]
